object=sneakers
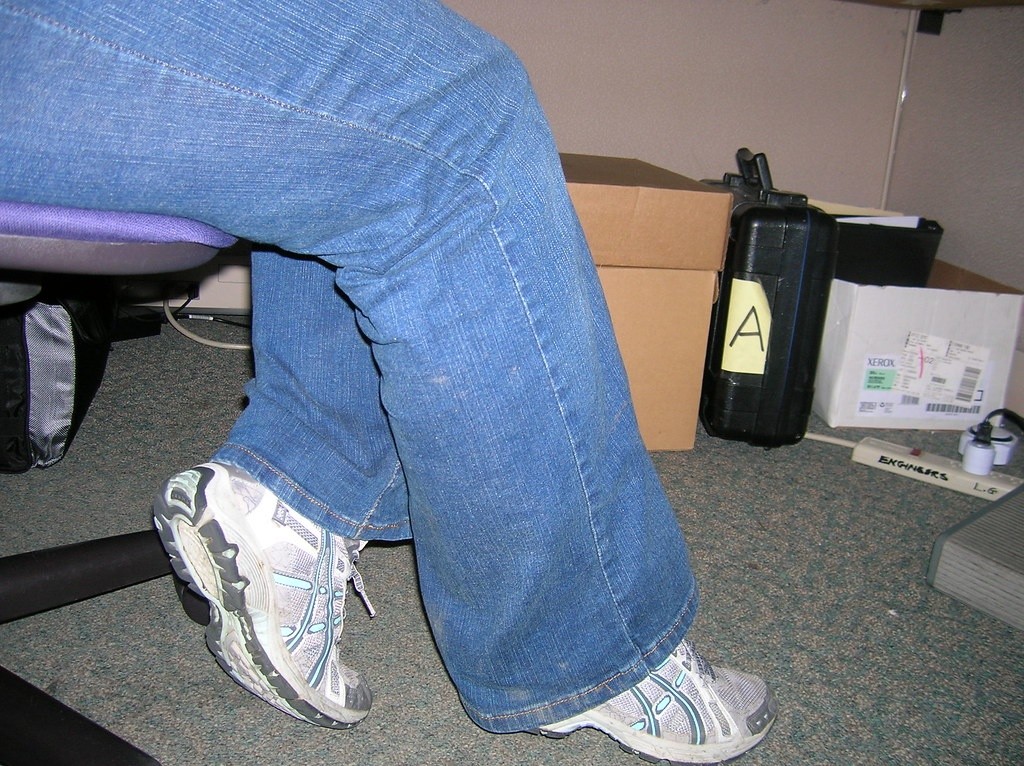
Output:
[153,461,376,731]
[524,638,778,766]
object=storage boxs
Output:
[560,152,733,451]
[809,199,1024,429]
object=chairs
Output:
[0,199,240,766]
